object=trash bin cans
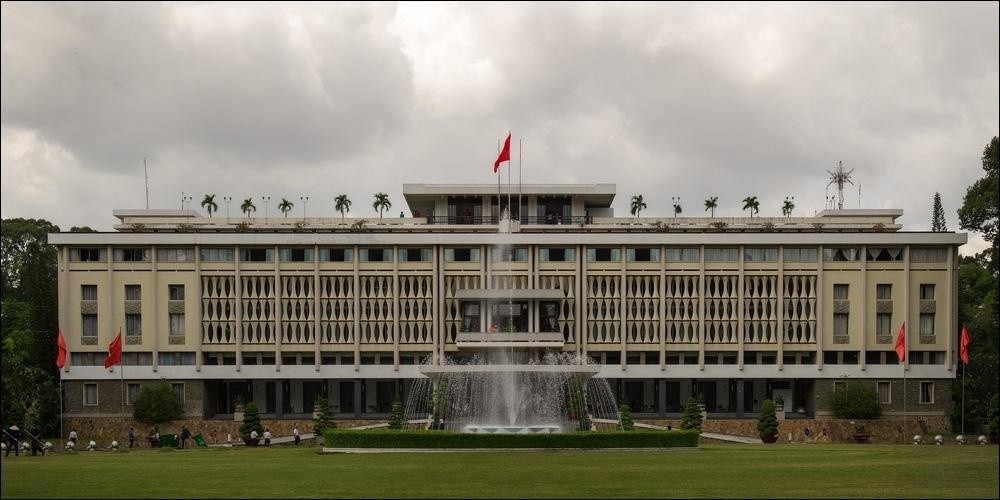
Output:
[159,434,178,446]
[194,433,208,448]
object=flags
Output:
[104,332,122,370]
[958,325,971,366]
[493,134,511,173]
[55,332,68,368]
[893,321,906,362]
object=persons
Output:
[250,428,259,447]
[293,426,301,445]
[68,428,78,445]
[399,212,404,218]
[149,429,156,448]
[177,425,191,449]
[128,427,135,448]
[31,423,45,456]
[4,425,20,457]
[263,428,272,446]
[155,430,161,448]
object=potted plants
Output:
[851,425,870,443]
[758,398,780,443]
[310,395,331,445]
[239,401,264,446]
